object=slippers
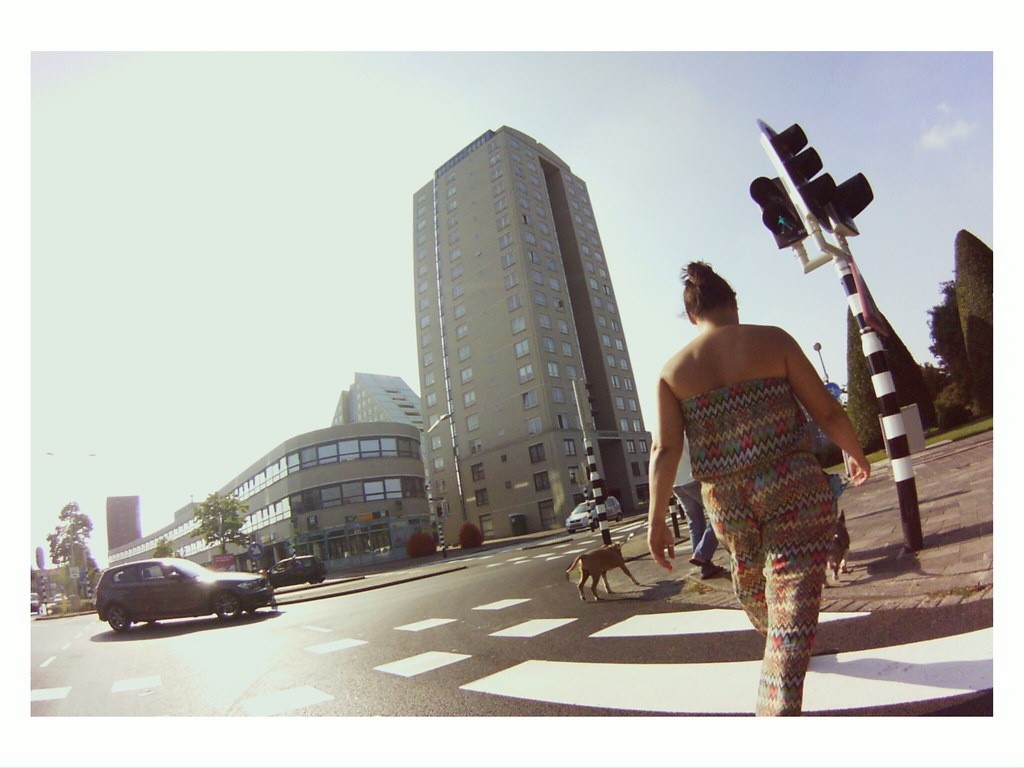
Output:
[690,558,716,570]
[701,565,724,580]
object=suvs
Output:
[264,555,326,590]
[94,558,273,632]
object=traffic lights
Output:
[756,118,837,231]
[826,173,875,237]
[751,176,808,250]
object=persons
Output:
[647,261,870,717]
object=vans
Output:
[565,496,623,534]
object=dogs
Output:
[565,540,640,603]
[823,508,850,589]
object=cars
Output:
[52,593,66,604]
[31,592,40,612]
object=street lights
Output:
[423,412,454,548]
[813,341,829,381]
[45,451,96,595]
